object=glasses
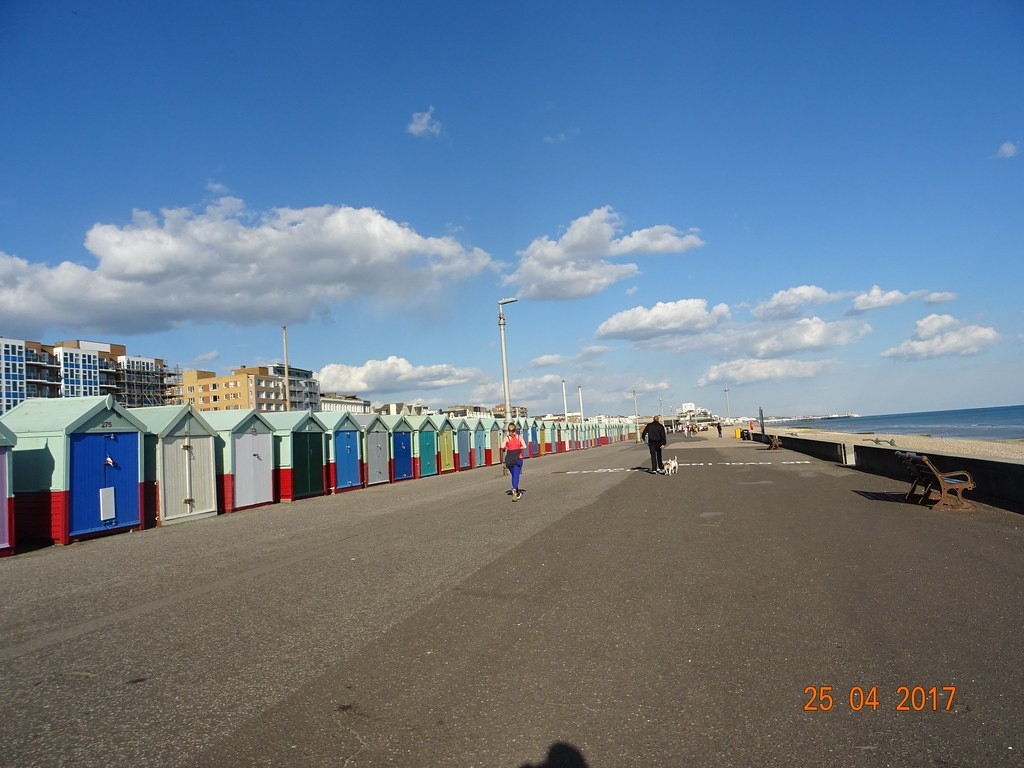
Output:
[508,429,516,433]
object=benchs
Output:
[895,451,979,510]
[765,434,783,451]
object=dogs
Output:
[661,456,678,476]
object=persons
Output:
[684,424,698,438]
[716,422,723,439]
[501,423,526,502]
[667,427,669,434]
[750,421,754,430]
[642,416,666,474]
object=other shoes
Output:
[653,470,656,473]
[512,490,517,502]
[660,469,664,473]
[518,492,522,498]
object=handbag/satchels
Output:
[505,451,518,467]
